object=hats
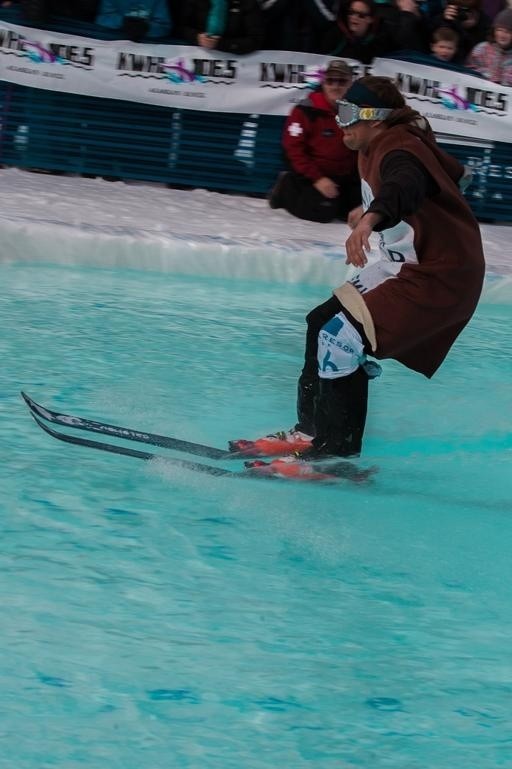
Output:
[326,59,351,75]
[491,7,511,30]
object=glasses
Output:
[324,77,352,88]
[334,98,396,128]
[346,8,373,19]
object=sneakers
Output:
[268,171,291,211]
[236,427,314,456]
[251,445,358,482]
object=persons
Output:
[231,76,487,479]
[269,60,362,223]
[1,0,511,222]
